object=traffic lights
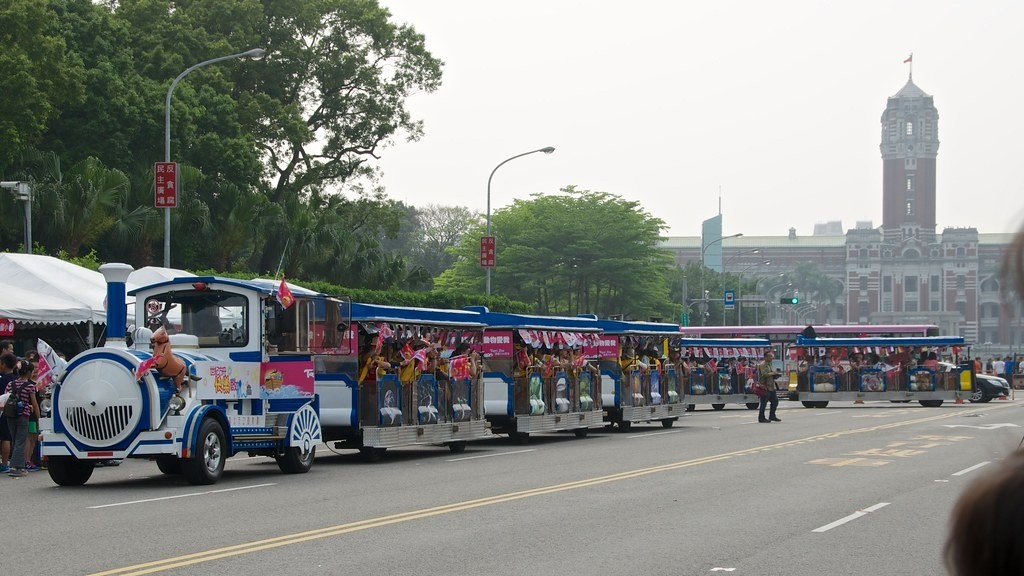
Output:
[781,298,797,304]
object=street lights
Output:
[975,271,995,344]
[485,144,557,291]
[163,45,266,271]
[700,234,819,325]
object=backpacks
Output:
[3,381,30,417]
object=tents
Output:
[0,252,320,349]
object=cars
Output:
[918,362,1010,404]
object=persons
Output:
[0,340,52,477]
[917,352,942,389]
[628,344,919,368]
[965,354,1024,389]
[513,343,581,371]
[758,350,783,423]
[942,447,1024,576]
[55,348,66,360]
[363,338,483,376]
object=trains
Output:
[36,266,965,495]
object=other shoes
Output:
[769,416,782,421]
[0,464,48,477]
[759,418,771,423]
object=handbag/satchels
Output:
[753,380,766,397]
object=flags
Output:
[35,338,68,392]
[449,354,470,382]
[399,342,415,360]
[735,361,744,376]
[275,276,294,309]
[703,358,719,377]
[374,325,391,354]
[414,348,425,371]
[808,345,963,357]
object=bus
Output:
[681,325,940,396]
